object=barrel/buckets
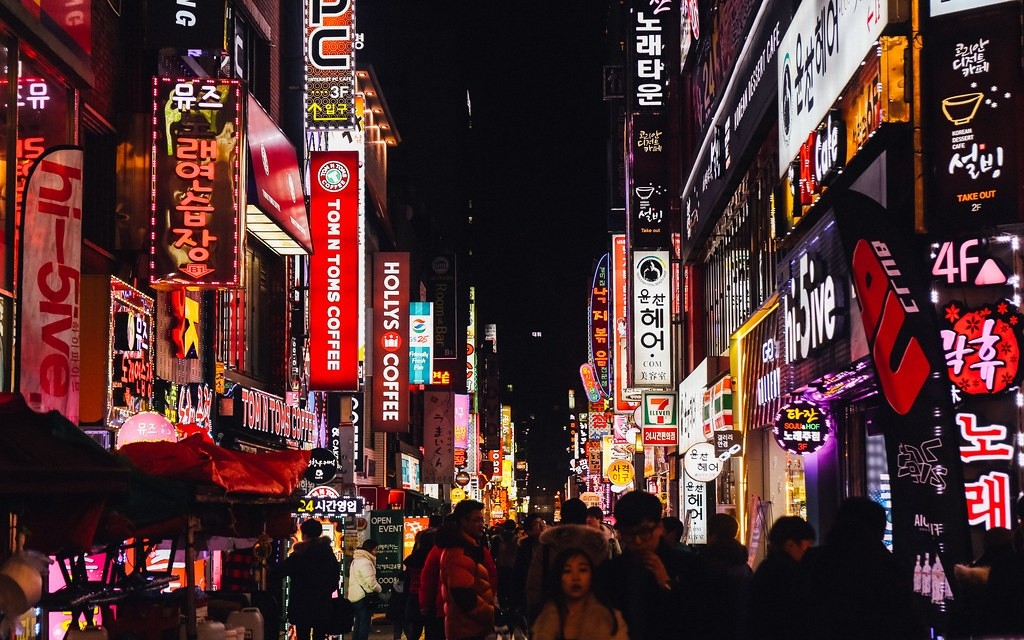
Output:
[225,629,237,640]
[231,625,245,640]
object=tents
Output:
[1,392,310,640]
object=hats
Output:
[540,524,610,567]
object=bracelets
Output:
[664,580,675,590]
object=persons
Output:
[401,490,1024,640]
[348,539,382,640]
[269,519,340,640]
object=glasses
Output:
[619,523,658,543]
[467,516,484,523]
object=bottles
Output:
[922,553,932,596]
[914,555,922,592]
[932,554,945,601]
[800,501,807,521]
[196,622,225,640]
[67,625,108,640]
[227,607,264,640]
